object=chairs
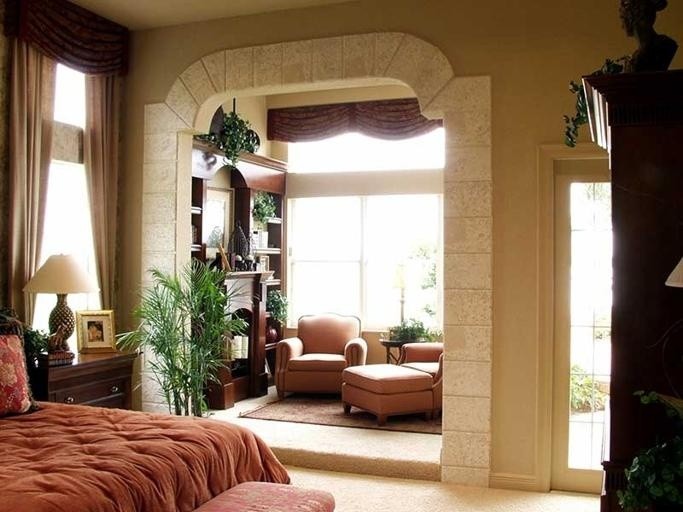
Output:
[276,312,444,420]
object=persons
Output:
[88,323,102,342]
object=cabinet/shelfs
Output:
[188,132,289,412]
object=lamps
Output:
[22,251,100,350]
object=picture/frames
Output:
[76,309,117,353]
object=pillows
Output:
[0,334,34,415]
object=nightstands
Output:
[37,348,145,410]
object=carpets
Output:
[238,395,442,434]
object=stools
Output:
[194,479,334,511]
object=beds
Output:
[0,313,271,511]
